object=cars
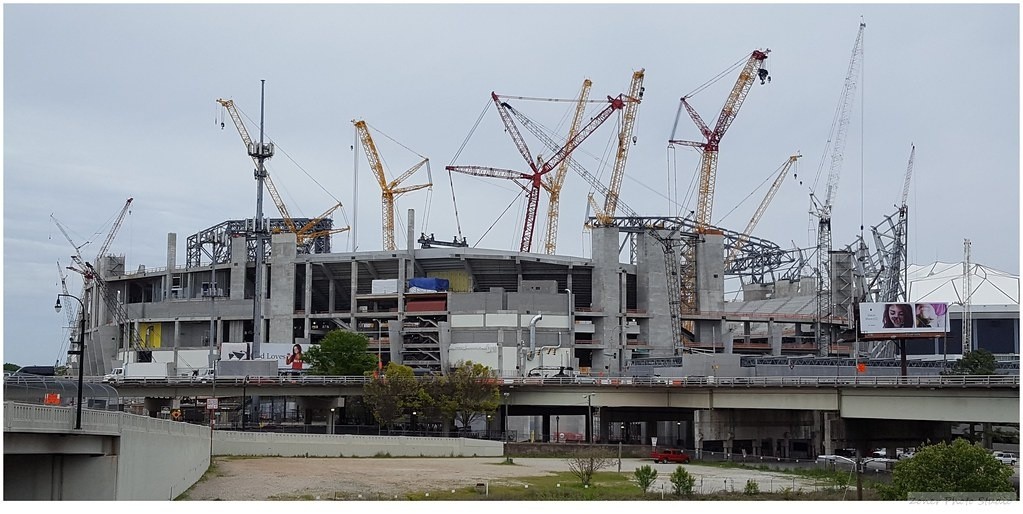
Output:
[814,445,915,469]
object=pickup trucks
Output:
[649,448,691,464]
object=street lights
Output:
[486,415,492,439]
[55,294,86,430]
[583,392,598,447]
[620,424,625,444]
[504,392,511,461]
[556,416,559,443]
[412,411,417,436]
[676,420,682,446]
[330,407,335,434]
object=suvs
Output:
[994,452,1018,466]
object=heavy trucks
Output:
[102,361,167,384]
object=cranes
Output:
[51,198,157,378]
[214,97,350,256]
[443,15,973,360]
[351,118,434,251]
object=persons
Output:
[882,304,913,328]
[916,304,946,328]
[286,344,303,369]
